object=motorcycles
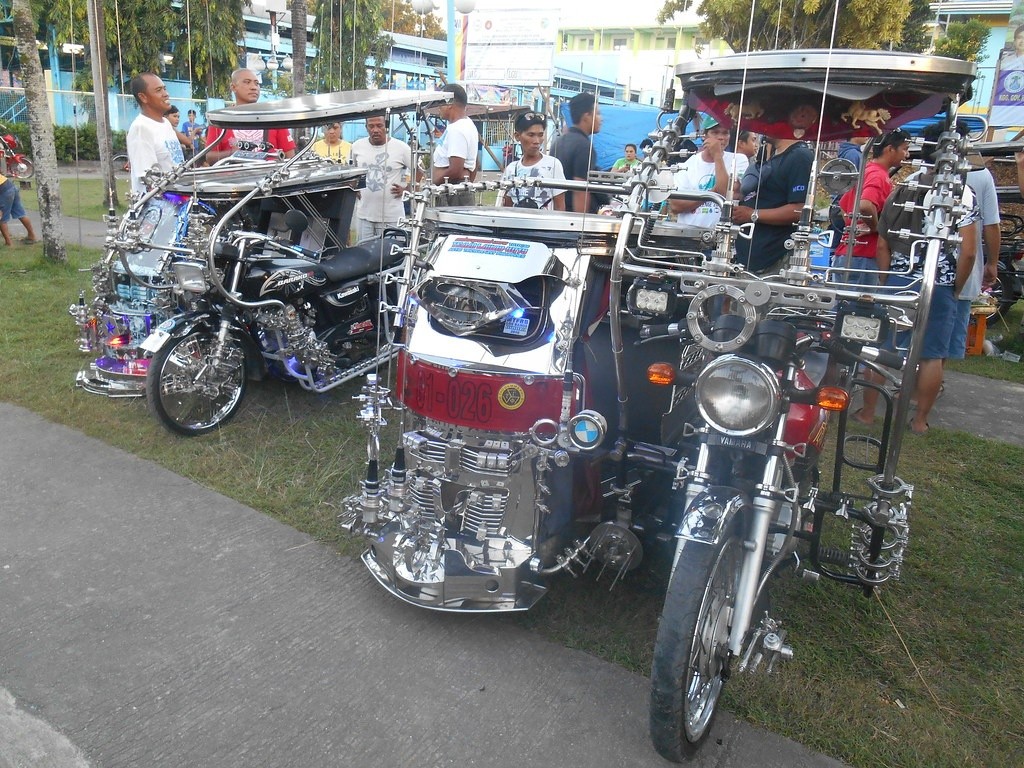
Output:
[0,124,36,180]
[60,45,1023,765]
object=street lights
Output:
[412,0,476,92]
[251,9,293,92]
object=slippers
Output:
[851,408,876,424]
[18,237,39,242]
[908,418,930,435]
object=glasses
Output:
[518,113,546,128]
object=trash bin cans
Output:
[960,298,995,355]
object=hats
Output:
[702,115,719,130]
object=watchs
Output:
[751,210,759,222]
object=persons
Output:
[729,130,758,158]
[205,69,295,165]
[127,73,185,212]
[312,122,352,163]
[502,142,512,168]
[831,123,1000,435]
[1001,25,1024,70]
[610,139,699,220]
[670,116,749,317]
[499,111,567,211]
[163,105,204,167]
[0,174,38,247]
[350,115,421,238]
[733,134,815,345]
[432,84,478,206]
[550,93,602,213]
